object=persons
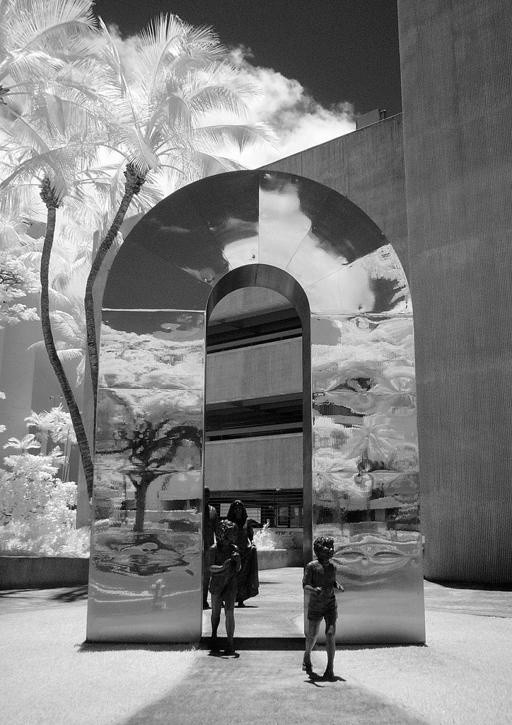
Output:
[203,483,222,608]
[226,498,263,610]
[205,518,244,660]
[301,533,344,683]
